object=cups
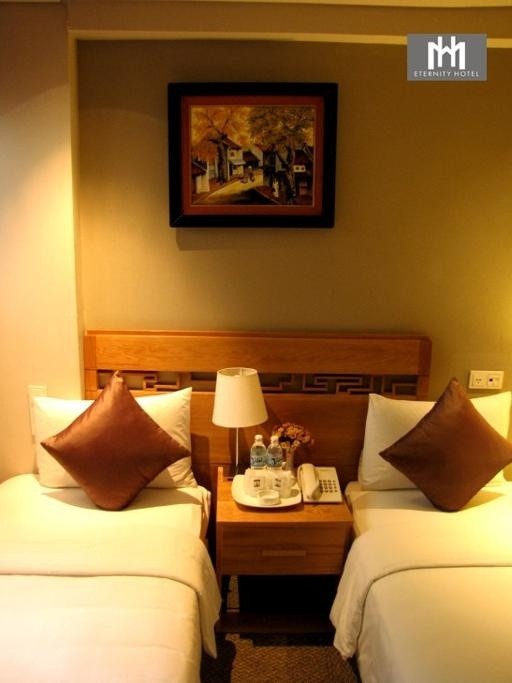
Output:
[273,471,296,498]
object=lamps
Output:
[211,367,269,479]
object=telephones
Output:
[297,463,343,504]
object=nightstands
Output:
[216,466,354,632]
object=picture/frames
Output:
[167,81,339,229]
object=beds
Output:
[1,474,212,683]
[345,482,511,682]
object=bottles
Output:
[267,435,283,491]
[249,433,267,494]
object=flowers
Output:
[270,423,315,453]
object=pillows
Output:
[40,370,192,511]
[357,393,512,491]
[378,376,512,512]
[33,387,199,488]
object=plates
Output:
[232,474,302,508]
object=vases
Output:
[285,451,295,476]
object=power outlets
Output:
[468,370,505,390]
